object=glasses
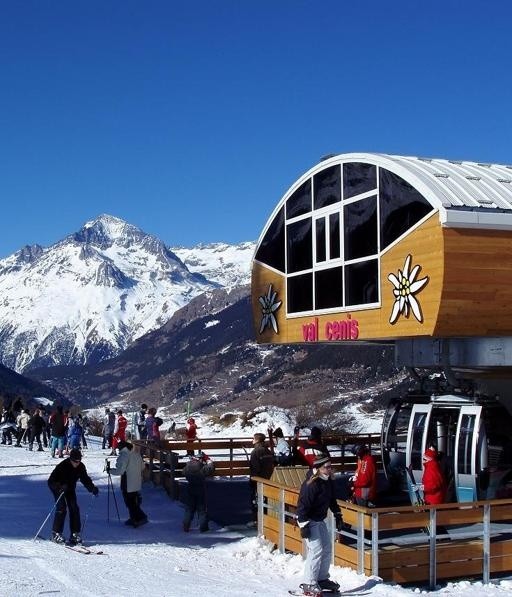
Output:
[74,460,80,462]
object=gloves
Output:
[336,516,343,530]
[300,520,311,538]
[107,467,110,473]
[419,484,424,490]
[201,451,210,461]
[92,486,99,496]
[410,484,418,491]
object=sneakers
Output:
[69,532,82,542]
[52,531,66,542]
[318,578,340,589]
[246,520,257,528]
[133,515,148,528]
[125,518,133,525]
[1,438,88,457]
[101,442,118,455]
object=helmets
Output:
[351,443,367,454]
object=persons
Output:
[349,443,377,507]
[184,452,210,531]
[272,427,292,466]
[246,432,275,527]
[411,446,451,541]
[47,447,99,544]
[183,417,196,457]
[292,426,330,465]
[1,403,90,458]
[103,403,164,454]
[106,440,148,526]
[296,454,344,592]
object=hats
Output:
[422,445,437,461]
[273,427,283,437]
[70,447,81,460]
[311,426,321,435]
[312,452,330,468]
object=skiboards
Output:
[403,463,440,543]
[267,426,278,467]
[291,426,299,466]
[288,584,372,597]
[35,533,103,554]
